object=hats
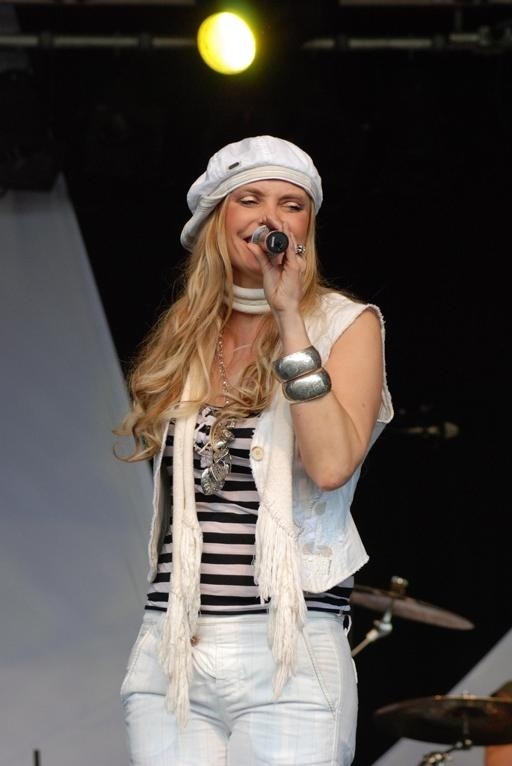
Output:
[180,135,323,253]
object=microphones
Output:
[251,224,289,254]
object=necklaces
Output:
[196,327,236,496]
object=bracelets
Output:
[269,345,323,384]
[281,370,332,404]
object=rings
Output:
[296,243,306,255]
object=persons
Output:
[111,135,397,764]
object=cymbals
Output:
[349,585,477,630]
[374,696,512,744]
